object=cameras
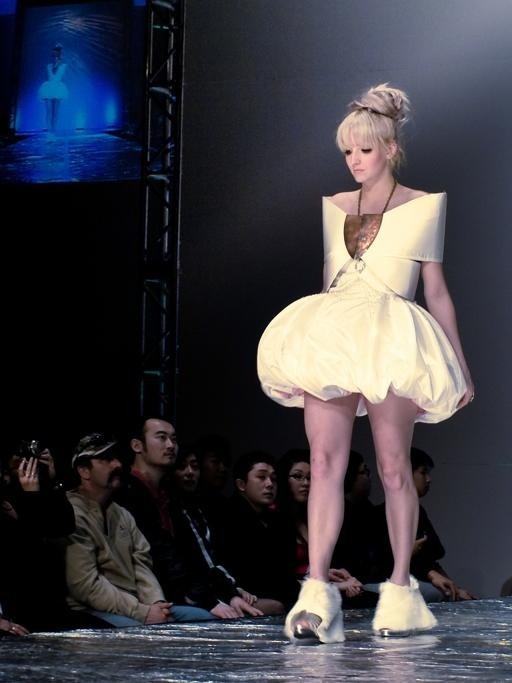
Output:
[16,438,45,460]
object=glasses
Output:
[289,474,310,480]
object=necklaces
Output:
[352,179,398,274]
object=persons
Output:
[39,37,71,133]
[256,80,476,645]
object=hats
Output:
[72,432,118,470]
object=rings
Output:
[470,395,473,402]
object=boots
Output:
[285,577,346,643]
[373,575,438,637]
